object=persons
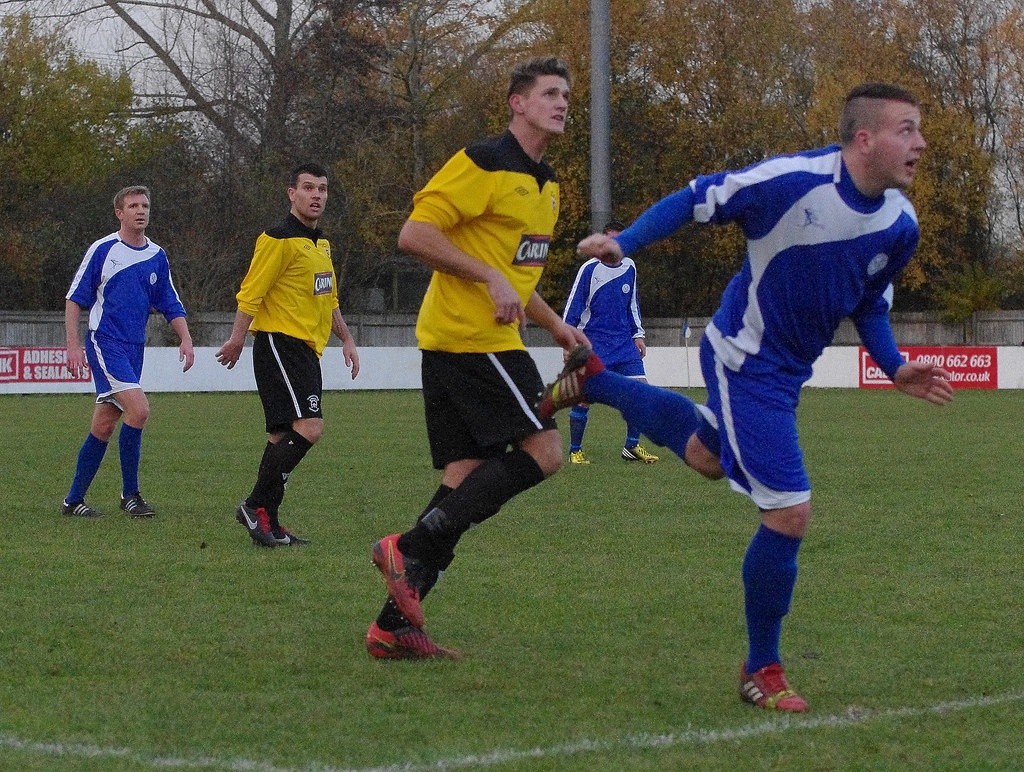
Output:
[215,162,361,547]
[62,186,194,518]
[536,83,953,710]
[365,60,592,659]
[563,224,660,465]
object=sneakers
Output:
[372,534,423,626]
[120,492,155,517]
[62,499,104,518]
[269,524,310,546]
[739,661,810,712]
[569,449,589,464]
[366,621,463,660]
[235,503,275,547]
[621,444,659,462]
[534,344,605,418]
